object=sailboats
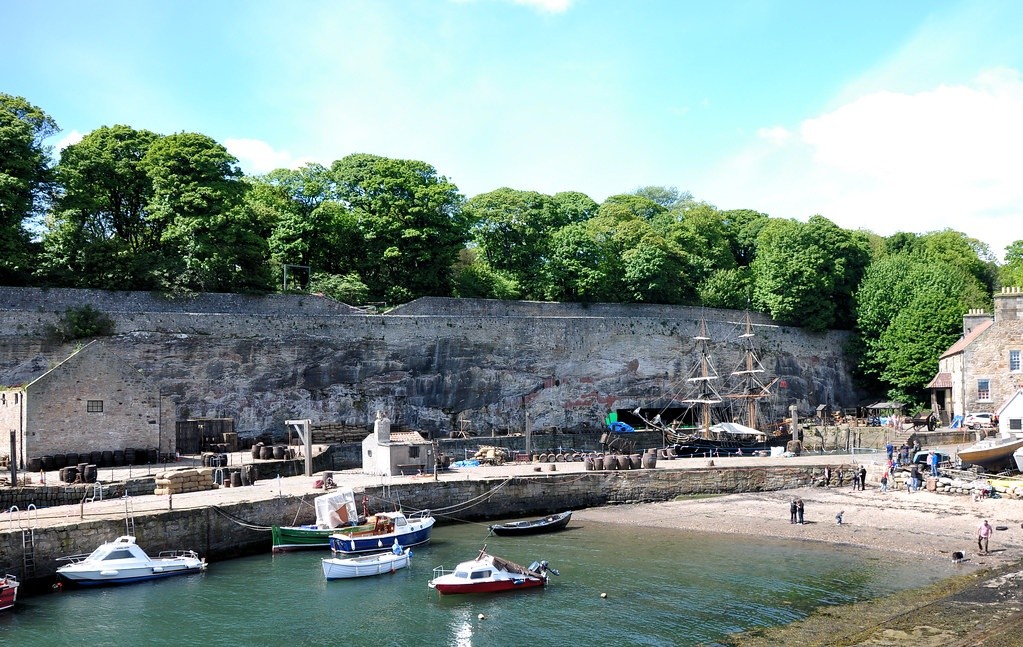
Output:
[629,299,805,457]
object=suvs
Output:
[964,412,995,430]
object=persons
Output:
[985,481,992,492]
[835,464,844,487]
[824,463,831,488]
[789,498,804,525]
[977,520,992,553]
[853,465,866,491]
[881,440,939,494]
[835,510,845,524]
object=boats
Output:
[427,554,546,595]
[320,547,414,582]
[328,508,436,554]
[489,509,573,536]
[270,488,376,553]
[54,535,208,586]
[956,433,1023,463]
[0,574,19,611]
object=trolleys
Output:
[904,411,937,433]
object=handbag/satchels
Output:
[607,421,634,433]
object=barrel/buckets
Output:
[539,453,610,462]
[230,465,255,487]
[27,448,157,472]
[584,448,675,470]
[203,437,232,453]
[58,463,98,483]
[201,451,228,468]
[251,444,288,460]
[323,472,333,490]
